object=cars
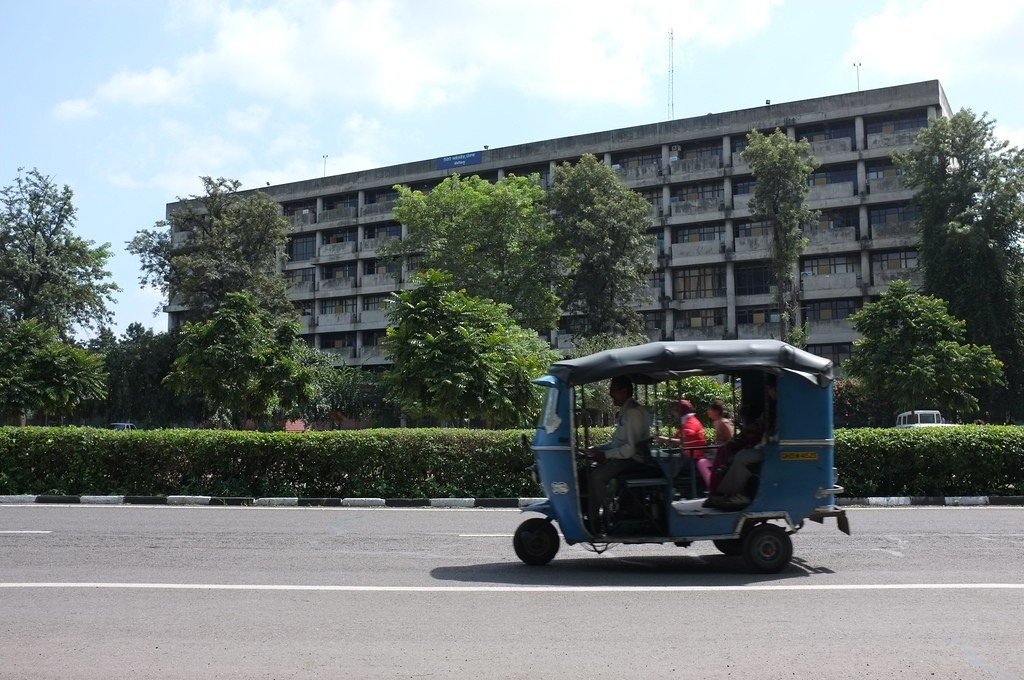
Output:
[106,423,136,431]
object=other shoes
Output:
[702,496,725,509]
[584,518,601,534]
[723,493,752,511]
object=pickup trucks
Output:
[896,410,959,427]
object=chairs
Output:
[614,479,668,516]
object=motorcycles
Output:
[513,337,849,574]
[648,419,714,500]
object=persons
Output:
[698,400,734,493]
[585,376,649,531]
[659,401,706,499]
